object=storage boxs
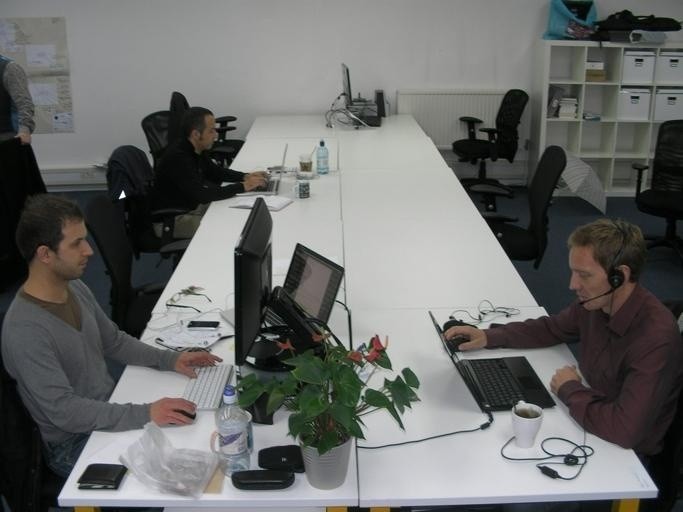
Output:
[620,88,650,122]
[657,52,683,86]
[622,51,655,84]
[654,90,683,120]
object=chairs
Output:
[141,111,171,176]
[83,195,193,336]
[105,146,195,274]
[0,273,67,512]
[469,146,567,269]
[630,119,682,249]
[170,90,243,160]
[636,299,679,512]
[452,90,529,203]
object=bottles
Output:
[316,141,329,175]
[216,385,249,478]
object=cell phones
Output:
[186,320,220,331]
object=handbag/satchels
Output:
[599,10,681,31]
[543,0,597,40]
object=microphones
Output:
[577,289,615,308]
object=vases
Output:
[299,435,352,490]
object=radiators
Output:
[395,90,530,151]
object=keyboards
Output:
[180,363,233,410]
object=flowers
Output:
[229,327,421,456]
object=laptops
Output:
[219,242,345,346]
[428,309,557,414]
[233,142,289,196]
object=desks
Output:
[350,305,660,512]
[343,166,538,306]
[228,111,449,169]
[151,169,346,314]
[56,310,358,512]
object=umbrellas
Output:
[549,155,607,216]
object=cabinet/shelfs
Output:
[527,40,683,198]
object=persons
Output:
[0,53,36,144]
[443,218,682,511]
[0,193,223,481]
[152,106,269,238]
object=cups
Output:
[511,403,544,449]
[210,410,254,458]
[293,180,310,199]
[300,160,312,172]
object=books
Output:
[558,98,578,119]
[228,195,293,212]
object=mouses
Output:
[168,408,197,426]
[447,336,470,351]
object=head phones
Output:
[607,216,629,290]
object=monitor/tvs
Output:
[341,62,353,107]
[233,197,274,366]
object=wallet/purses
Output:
[77,464,128,489]
